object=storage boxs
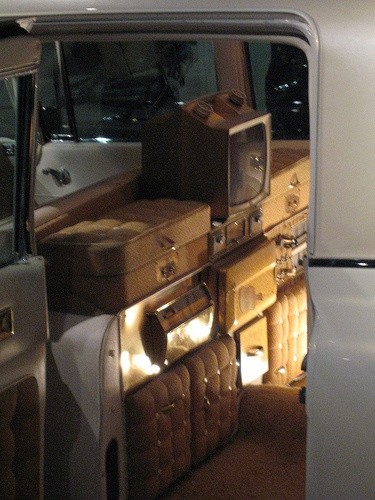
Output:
[257,149,314,232]
[34,198,210,314]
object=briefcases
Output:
[39,199,212,312]
